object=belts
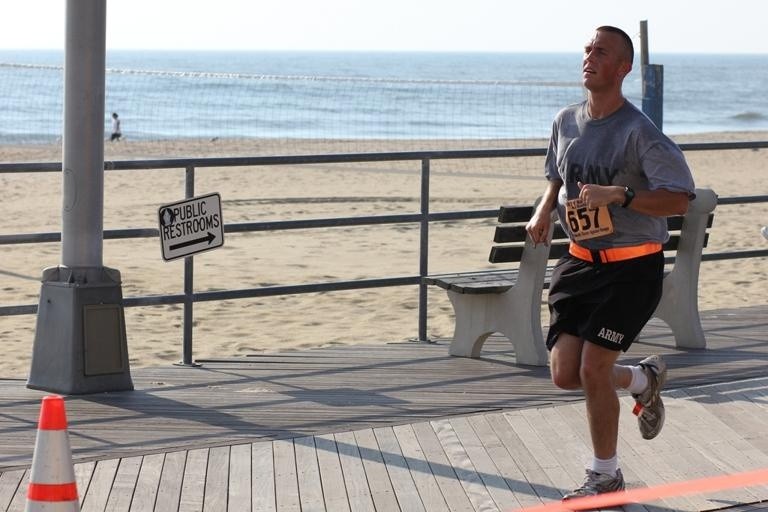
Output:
[568,240,661,263]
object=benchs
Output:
[421,186,721,365]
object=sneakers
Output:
[562,467,625,503]
[631,355,666,440]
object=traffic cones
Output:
[23,394,83,511]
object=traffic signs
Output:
[158,192,227,262]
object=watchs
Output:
[618,185,636,210]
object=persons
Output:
[110,112,122,140]
[524,25,695,500]
